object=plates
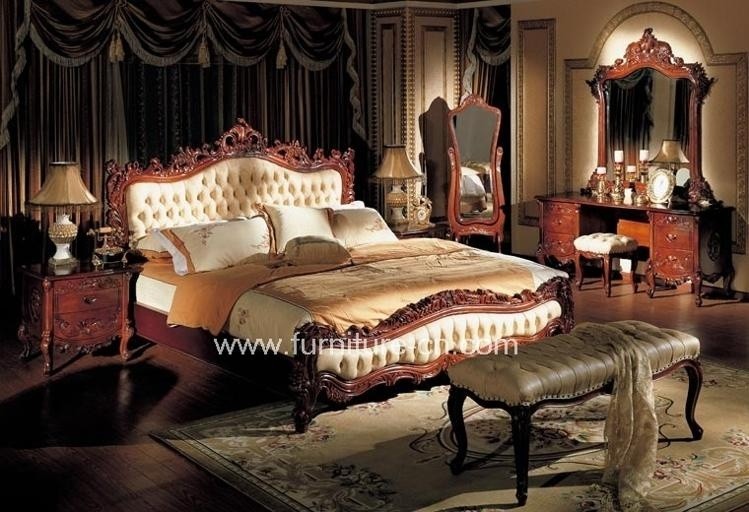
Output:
[646,167,676,204]
[676,167,690,187]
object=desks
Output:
[536,193,736,306]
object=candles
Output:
[614,151,623,162]
[597,167,606,174]
[640,150,648,161]
[627,165,636,172]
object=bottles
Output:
[623,188,633,206]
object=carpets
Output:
[148,359,749,512]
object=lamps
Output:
[371,145,422,223]
[650,140,688,169]
[27,161,96,266]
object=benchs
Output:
[446,318,705,507]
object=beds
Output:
[460,167,487,213]
[104,115,576,435]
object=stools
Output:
[574,232,638,297]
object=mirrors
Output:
[447,96,506,252]
[648,169,675,211]
[587,28,713,204]
[672,166,692,191]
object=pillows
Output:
[135,201,399,266]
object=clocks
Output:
[412,196,432,228]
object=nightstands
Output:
[18,266,133,376]
[388,219,434,239]
